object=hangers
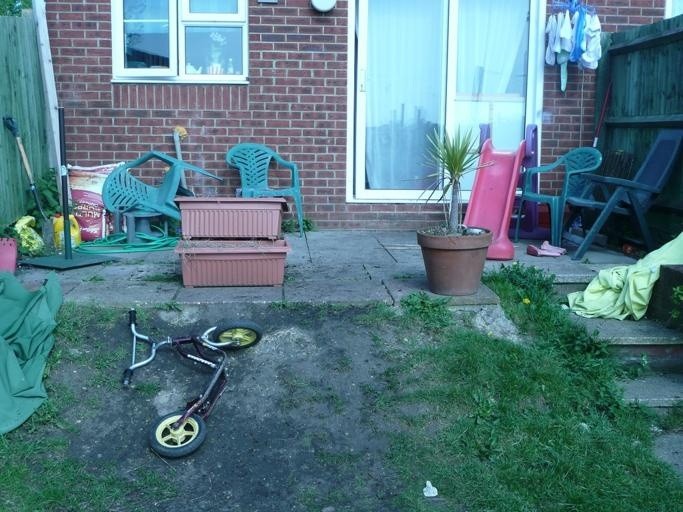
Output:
[550,0,597,16]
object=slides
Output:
[463,140,525,261]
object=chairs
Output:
[225,142,304,238]
[567,128,682,260]
[102,151,222,250]
[513,146,603,248]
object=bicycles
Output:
[117,304,260,462]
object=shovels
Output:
[3,116,53,248]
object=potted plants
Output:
[417,124,493,297]
